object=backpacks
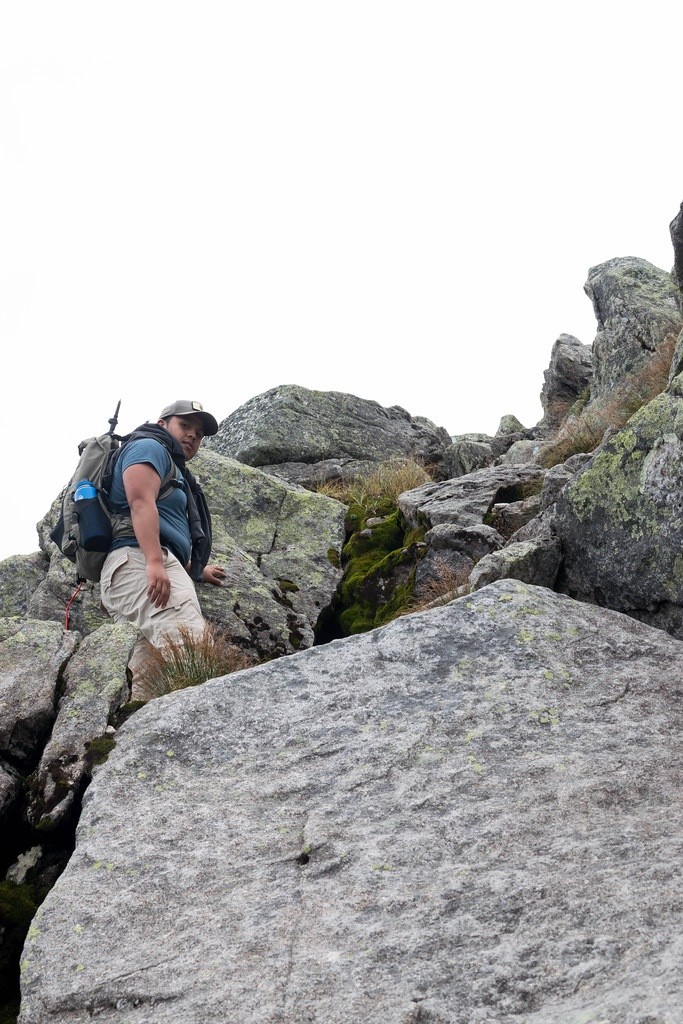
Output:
[50,432,186,583]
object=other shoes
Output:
[107,707,121,734]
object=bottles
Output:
[73,481,110,550]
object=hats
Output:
[159,400,218,436]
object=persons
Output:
[98,398,227,705]
